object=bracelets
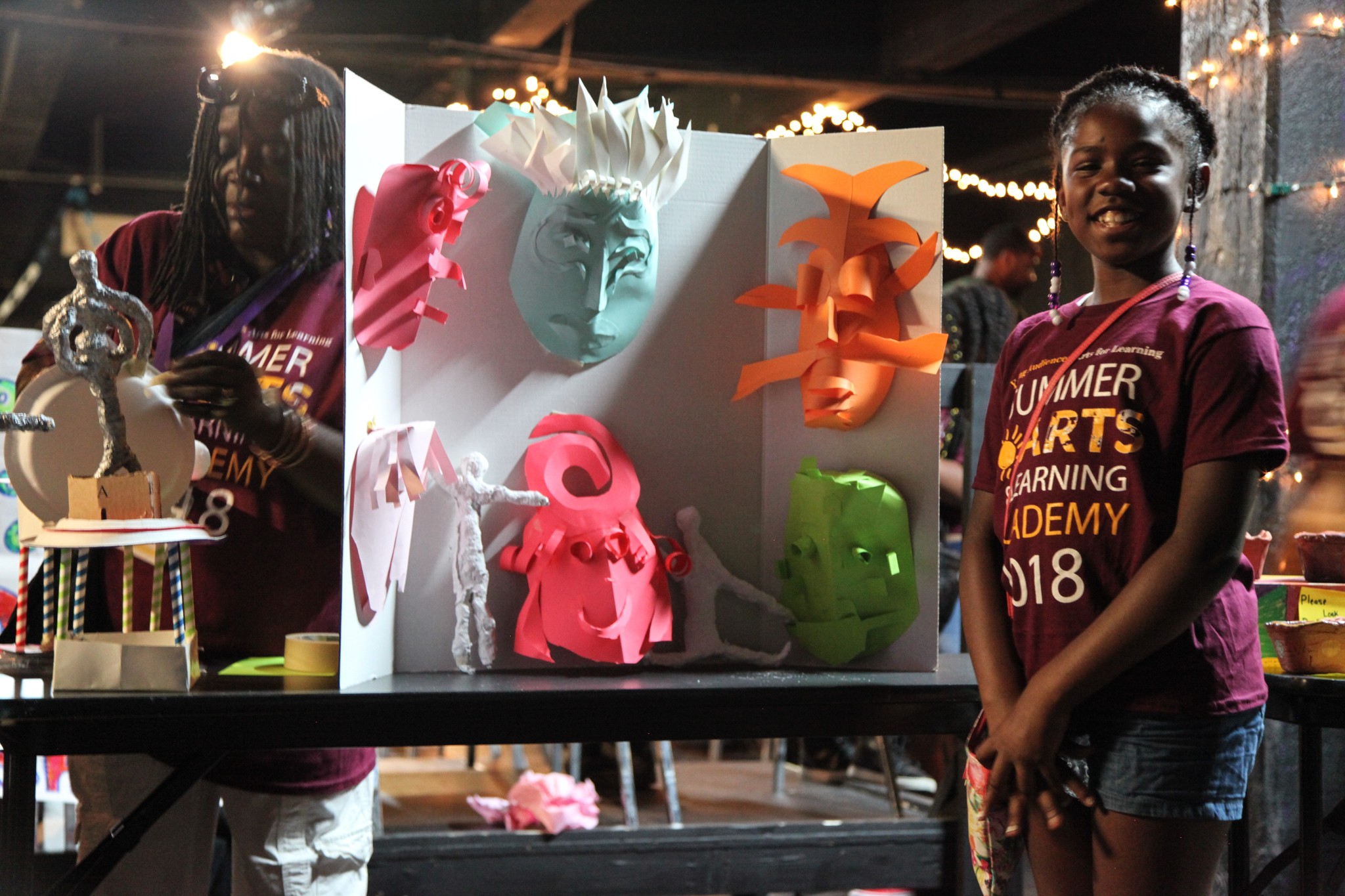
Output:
[264,400,318,468]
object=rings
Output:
[218,387,234,407]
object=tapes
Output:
[282,632,340,674]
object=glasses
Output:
[196,65,330,117]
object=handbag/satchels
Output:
[963,707,1029,895]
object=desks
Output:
[1223,666,1345,895]
[0,657,1007,896]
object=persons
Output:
[14,41,379,896]
[951,61,1294,891]
[946,218,1042,366]
